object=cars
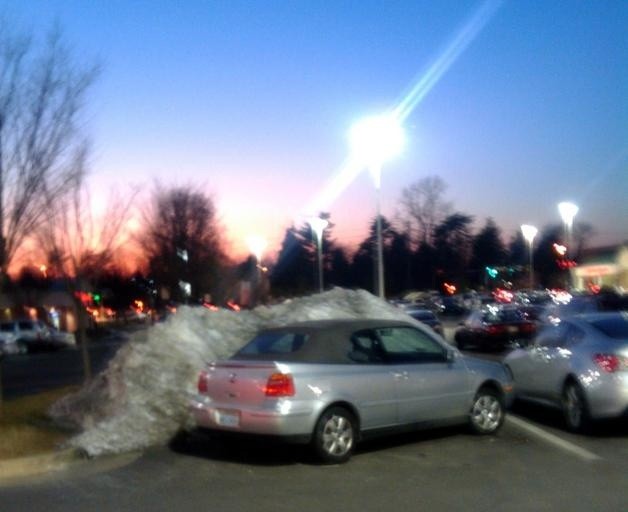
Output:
[0,316,76,357]
[190,318,517,466]
[454,304,537,352]
[502,311,627,433]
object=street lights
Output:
[361,130,386,299]
[558,201,579,291]
[520,223,538,291]
[307,216,329,292]
